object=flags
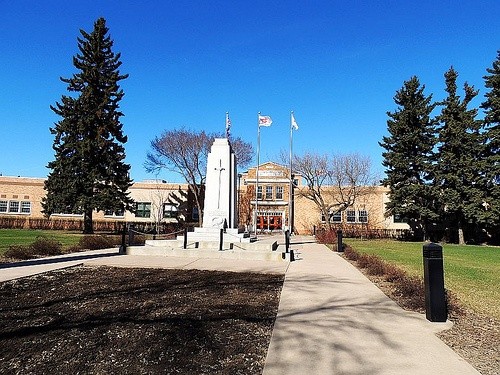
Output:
[292,114,298,130]
[259,115,272,126]
[226,116,231,137]
